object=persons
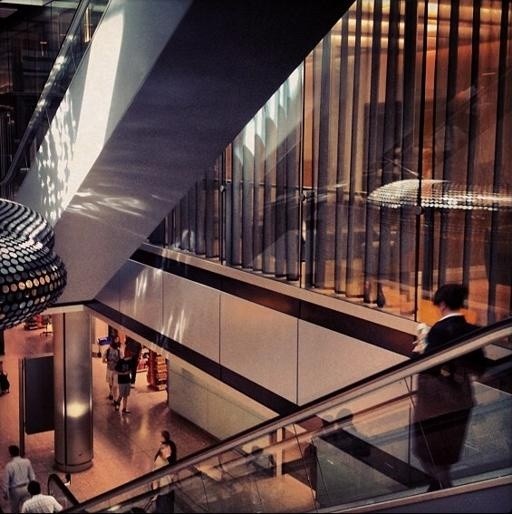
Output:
[102,334,142,415]
[151,429,176,514]
[21,481,63,514]
[302,409,374,507]
[410,284,487,492]
[1,445,36,513]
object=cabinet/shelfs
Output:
[108,324,168,391]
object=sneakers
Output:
[106,394,132,415]
